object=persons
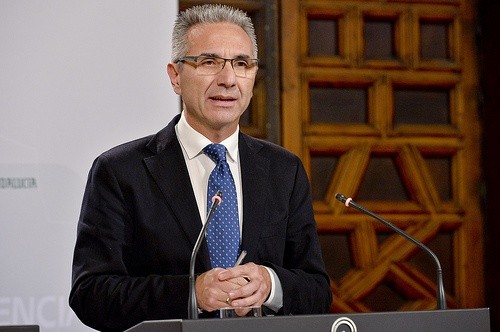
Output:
[69,4,334,332]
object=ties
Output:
[201,141,243,270]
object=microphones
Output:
[188,191,223,321]
[335,194,447,310]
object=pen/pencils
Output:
[232,250,247,267]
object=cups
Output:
[220,306,261,319]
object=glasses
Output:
[174,55,259,77]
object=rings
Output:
[226,298,230,303]
